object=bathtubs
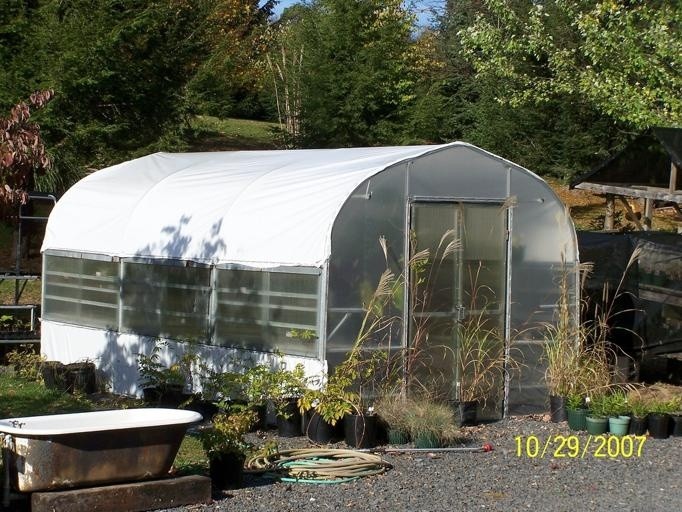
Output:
[0,405,203,493]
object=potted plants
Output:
[35,352,99,401]
[540,246,682,440]
[135,228,518,448]
[178,390,266,487]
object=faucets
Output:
[19,422,26,429]
[10,420,19,427]
